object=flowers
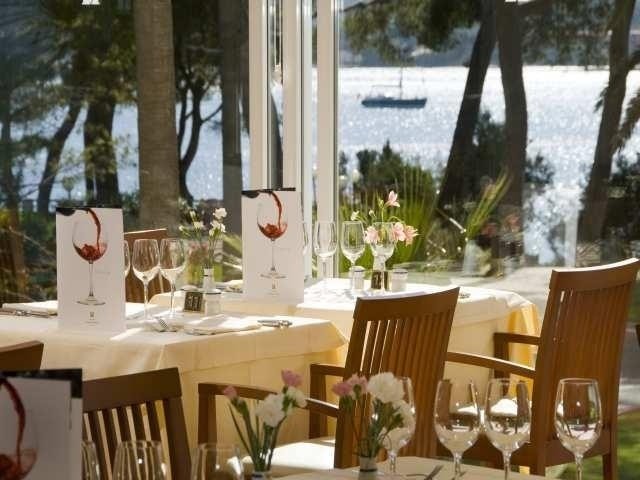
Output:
[349,189,420,248]
[179,206,232,270]
[331,371,417,469]
[221,368,309,471]
[472,176,521,239]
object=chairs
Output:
[0,339,46,380]
[120,228,177,304]
[439,256,640,480]
[79,366,192,480]
[196,286,461,480]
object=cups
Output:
[0,393,38,480]
[108,440,247,479]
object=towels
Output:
[0,300,60,318]
[184,313,266,336]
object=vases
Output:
[251,471,275,480]
[202,269,218,289]
[356,455,380,480]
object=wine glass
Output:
[364,375,606,480]
[72,220,110,307]
[121,238,184,327]
[313,217,394,300]
[256,203,290,281]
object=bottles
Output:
[390,267,408,293]
[242,190,273,199]
[56,207,91,218]
[204,287,221,316]
